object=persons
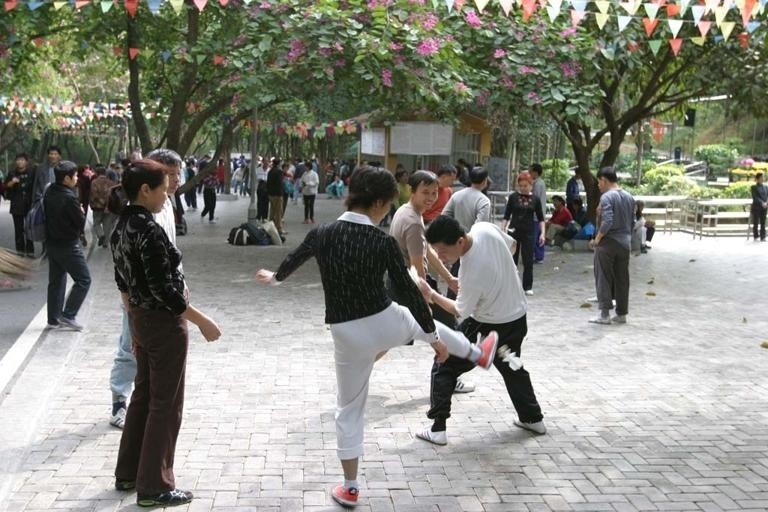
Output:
[112,160,220,507]
[252,155,325,234]
[110,150,188,429]
[440,165,491,309]
[35,145,61,196]
[527,165,547,265]
[321,151,494,205]
[299,163,319,222]
[256,166,498,507]
[501,172,545,294]
[567,168,582,200]
[751,173,768,242]
[566,198,586,235]
[89,166,112,249]
[590,169,635,325]
[2,152,36,258]
[408,213,547,445]
[388,170,474,390]
[183,150,218,223]
[217,153,253,197]
[540,197,572,250]
[631,200,656,252]
[100,160,119,183]
[70,165,93,248]
[44,161,92,331]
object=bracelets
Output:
[430,289,435,302]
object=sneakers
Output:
[612,314,627,323]
[525,290,534,296]
[109,408,127,428]
[331,485,359,508]
[513,417,548,434]
[588,315,611,325]
[641,245,646,254]
[415,427,448,445]
[537,260,543,264]
[137,489,193,507]
[115,478,136,490]
[98,236,106,246]
[476,331,498,370]
[455,378,475,393]
[80,235,87,246]
[57,314,83,331]
[47,323,67,329]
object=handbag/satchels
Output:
[260,217,282,245]
[1,170,16,201]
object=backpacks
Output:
[24,183,51,264]
